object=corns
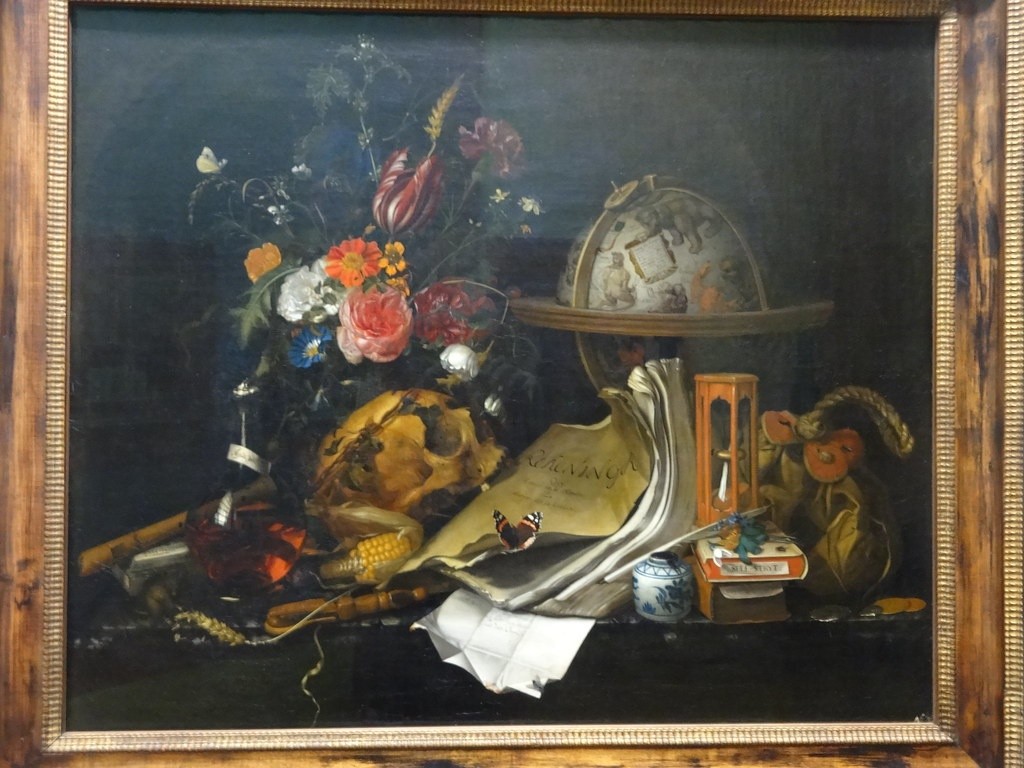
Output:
[318,533,413,584]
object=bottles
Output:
[632,552,694,621]
[186,379,306,590]
